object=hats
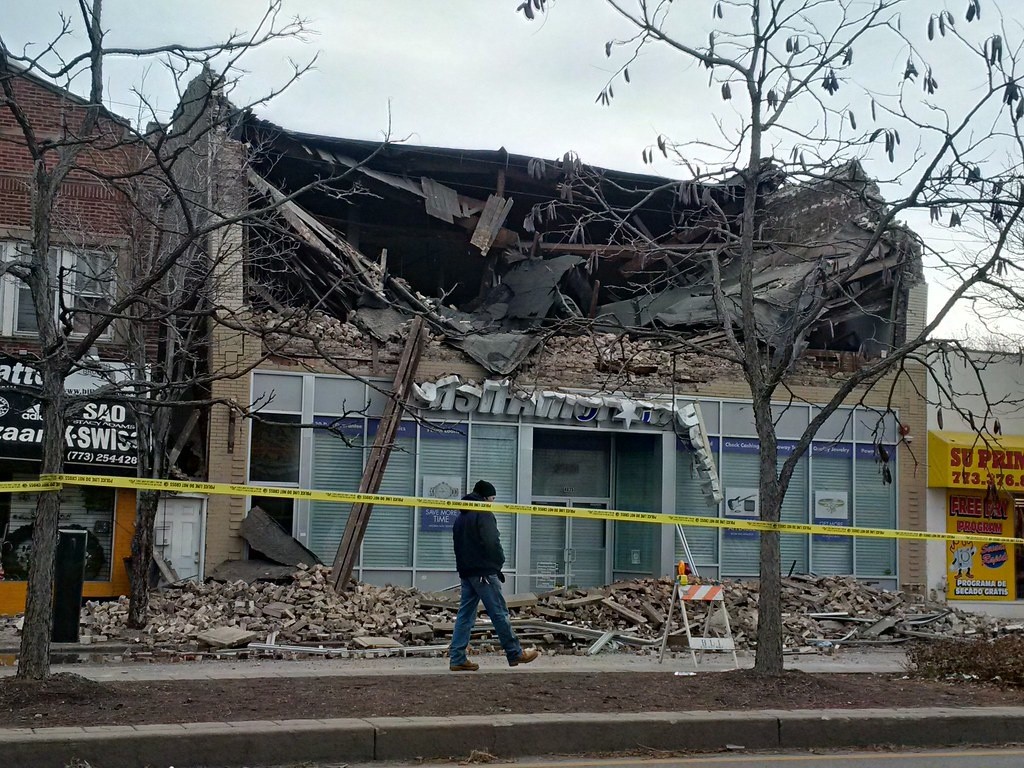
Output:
[473,480,496,497]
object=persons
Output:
[448,478,539,671]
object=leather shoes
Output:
[450,659,479,672]
[509,651,538,666]
